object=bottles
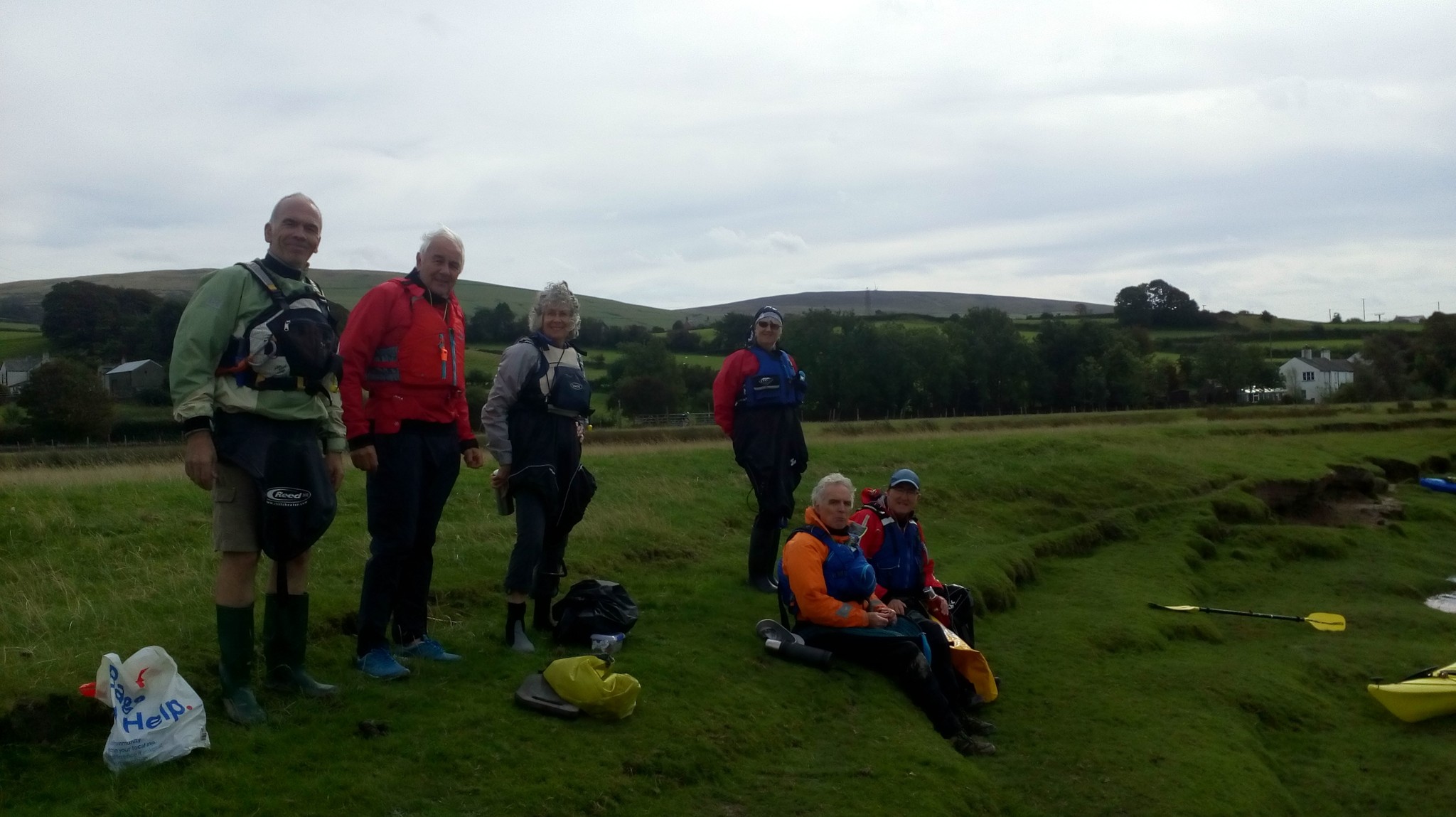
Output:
[923,586,951,627]
[493,468,515,516]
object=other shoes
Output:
[961,718,993,738]
[950,735,997,757]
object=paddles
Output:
[1146,598,1347,634]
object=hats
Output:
[887,467,921,493]
[756,619,803,646]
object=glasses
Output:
[758,321,783,331]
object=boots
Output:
[533,597,558,633]
[262,591,334,697]
[214,604,267,726]
[504,599,538,653]
[749,526,780,594]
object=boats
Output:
[1420,474,1456,496]
[1367,660,1456,725]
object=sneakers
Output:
[399,640,464,664]
[352,653,413,681]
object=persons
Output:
[847,468,1001,706]
[481,283,591,650]
[778,471,996,755]
[339,228,483,680]
[168,194,346,732]
[712,306,805,592]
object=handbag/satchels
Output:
[95,645,211,774]
[543,658,637,716]
